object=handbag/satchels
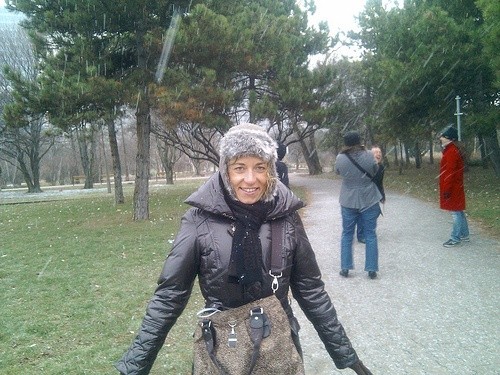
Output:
[193,294,305,375]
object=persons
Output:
[275,139,290,190]
[357,146,386,244]
[333,130,383,279]
[113,122,373,375]
[439,128,471,248]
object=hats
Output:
[219,123,278,201]
[441,127,458,140]
[344,131,361,146]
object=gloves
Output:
[350,360,373,375]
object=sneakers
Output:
[461,237,470,242]
[444,239,462,247]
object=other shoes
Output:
[360,238,366,243]
[339,269,349,278]
[368,271,378,279]
